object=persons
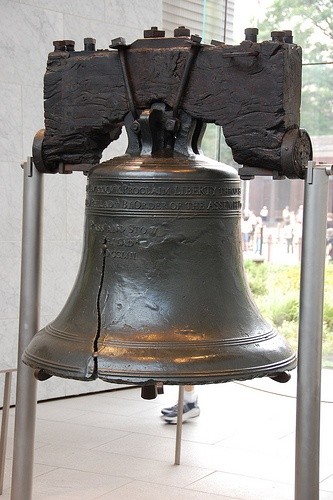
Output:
[161,385,201,425]
[240,206,333,262]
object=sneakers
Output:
[161,404,179,414]
[163,403,200,423]
